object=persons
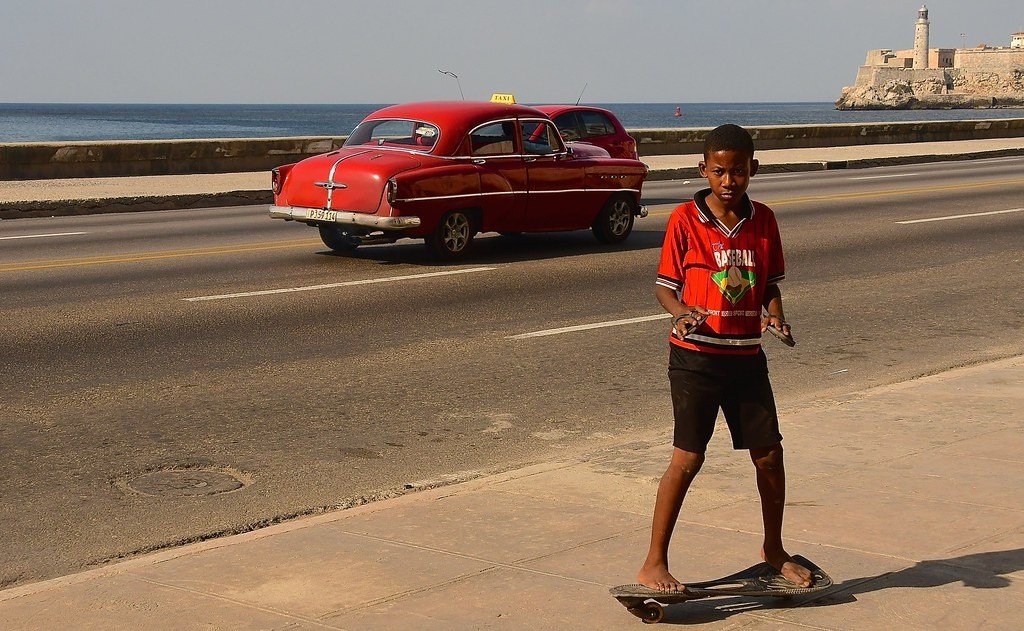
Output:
[635,125,814,593]
[502,123,551,156]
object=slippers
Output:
[671,311,708,334]
[760,314,796,347]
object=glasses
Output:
[521,125,524,131]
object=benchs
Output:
[417,134,514,153]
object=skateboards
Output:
[608,552,836,625]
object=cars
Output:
[527,104,639,162]
[269,100,649,259]
